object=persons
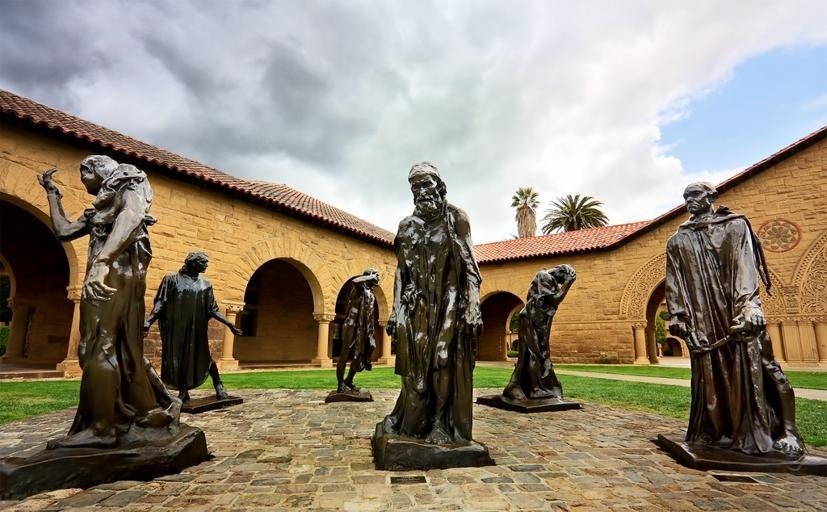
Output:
[334,267,382,395]
[377,158,487,445]
[141,248,246,405]
[502,262,579,402]
[662,180,811,460]
[33,152,183,452]
